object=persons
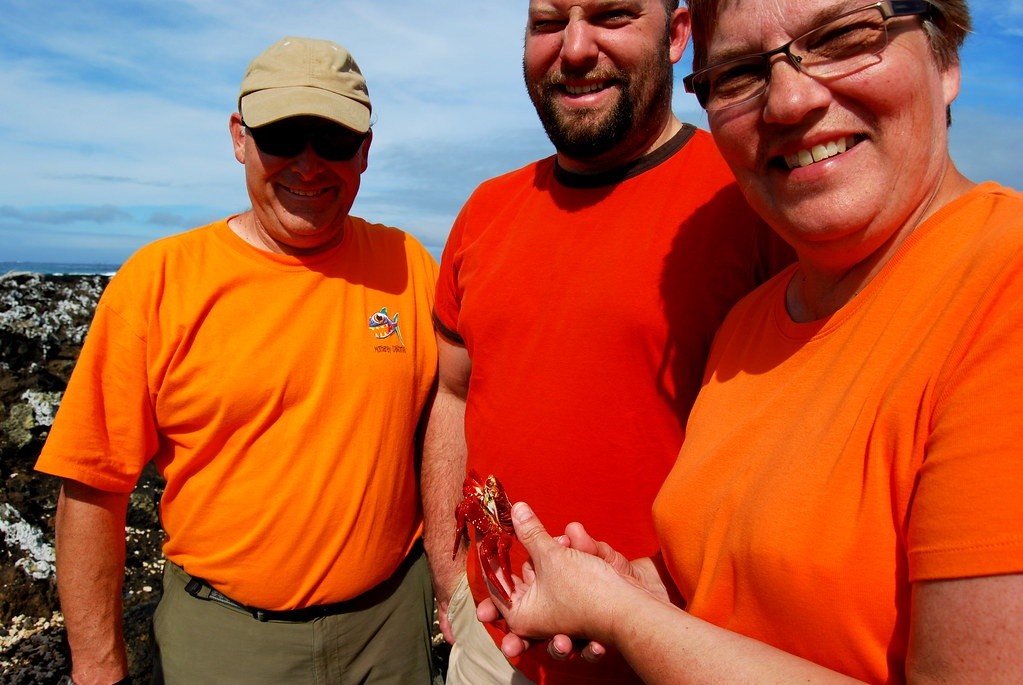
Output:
[32,36,442,685]
[420,2,799,685]
[477,2,1023,685]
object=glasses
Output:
[243,122,365,161]
[682,0,950,112]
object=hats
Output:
[236,36,372,133]
